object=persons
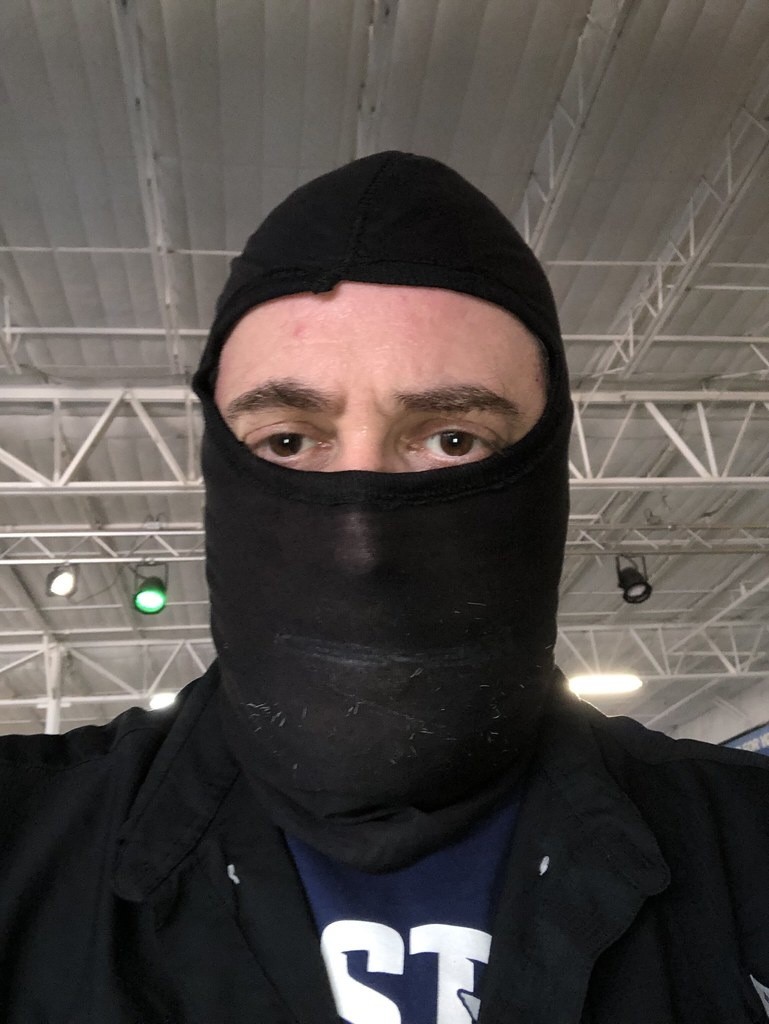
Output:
[0,150,767,1024]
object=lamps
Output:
[132,561,170,613]
[614,555,652,603]
[47,566,80,599]
